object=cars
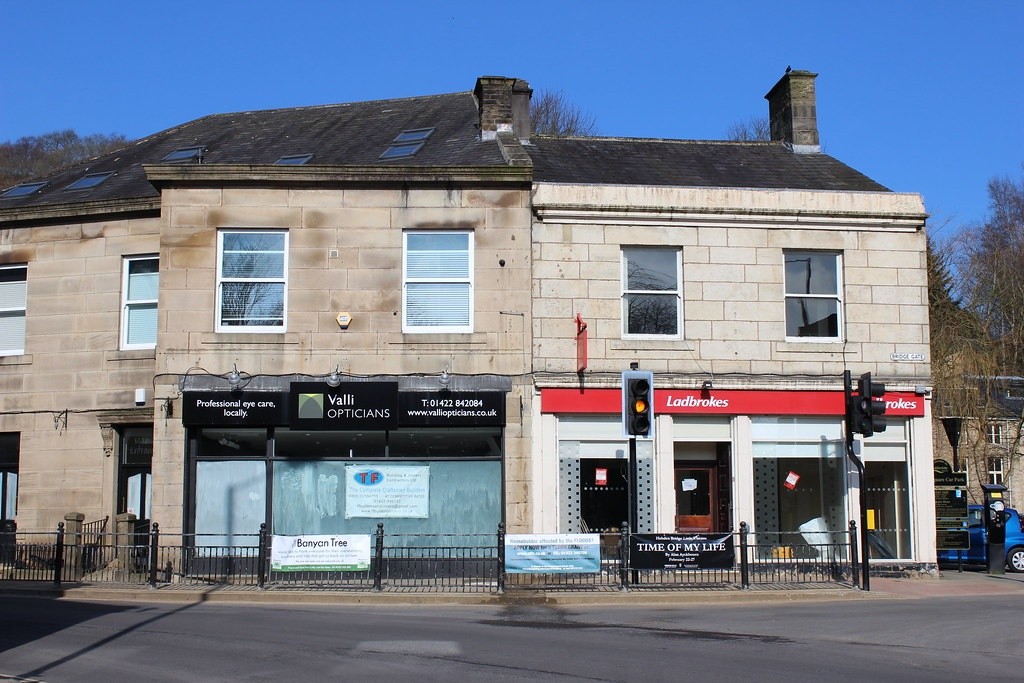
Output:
[936,503,1024,573]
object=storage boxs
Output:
[773,546,794,559]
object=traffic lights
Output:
[857,372,886,438]
[628,378,651,435]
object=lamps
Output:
[439,365,452,393]
[229,363,253,393]
[326,364,342,388]
[52,408,68,437]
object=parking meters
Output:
[981,484,1008,574]
